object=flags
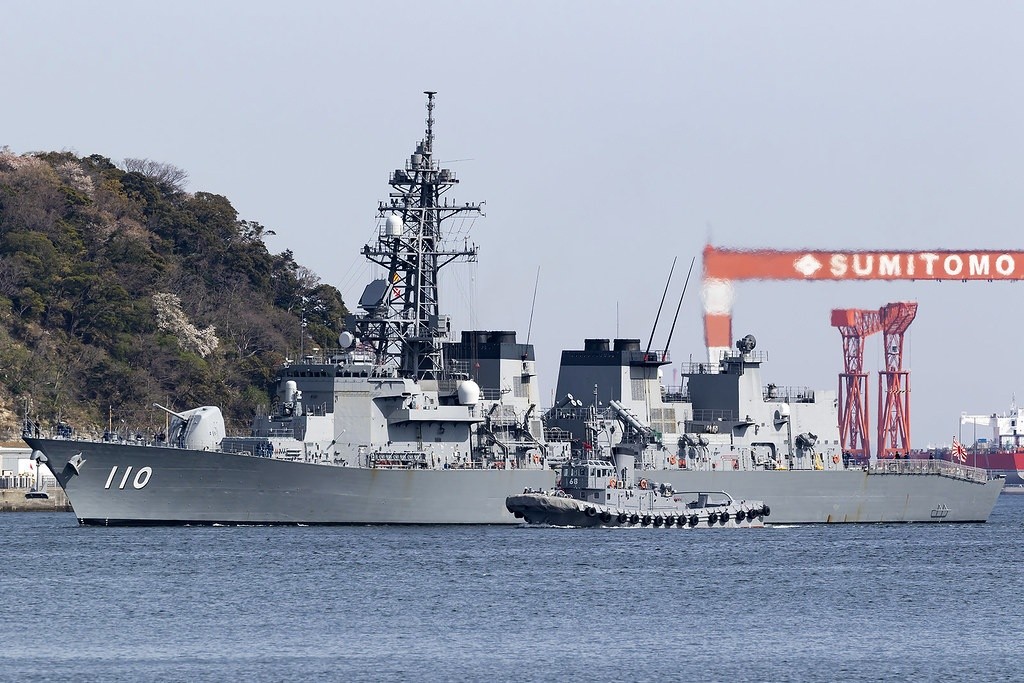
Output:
[951,435,967,462]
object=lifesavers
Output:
[710,513,717,523]
[585,507,596,516]
[630,514,639,523]
[690,515,698,525]
[666,516,674,525]
[764,506,770,515]
[655,516,663,525]
[640,479,647,489]
[600,512,611,522]
[619,513,627,522]
[738,511,745,520]
[722,512,729,521]
[679,459,686,466]
[671,455,676,464]
[679,515,686,525]
[833,456,840,464]
[535,456,539,464]
[749,509,757,518]
[643,515,651,525]
[610,479,616,486]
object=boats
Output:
[505,334,1007,529]
[22,90,692,524]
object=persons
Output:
[842,450,862,466]
[136,432,142,441]
[57,422,72,438]
[256,441,274,458]
[24,418,41,440]
[102,428,110,441]
[886,452,911,468]
[929,453,934,471]
[699,363,704,374]
[154,431,165,443]
